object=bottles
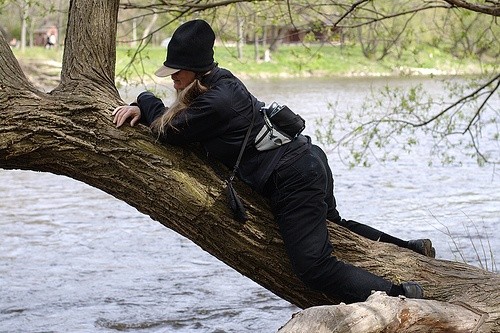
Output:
[267,102,282,118]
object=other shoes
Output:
[401,282,424,298]
[408,238,436,258]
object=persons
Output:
[3,24,57,49]
[111,19,436,305]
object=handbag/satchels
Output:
[243,105,305,155]
[225,181,249,222]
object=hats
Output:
[154,19,216,78]
